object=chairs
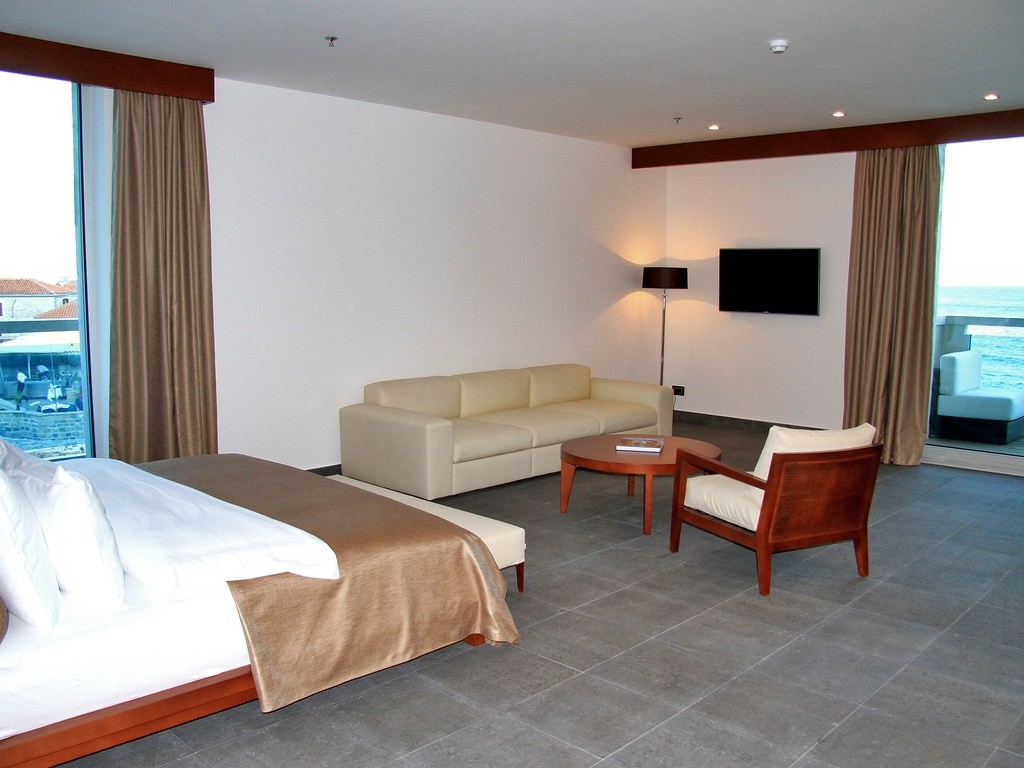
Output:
[667,441,886,597]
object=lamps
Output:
[642,267,689,385]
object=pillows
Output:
[0,436,130,612]
[0,468,63,632]
[743,423,877,509]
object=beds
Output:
[0,454,520,768]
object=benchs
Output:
[326,474,526,593]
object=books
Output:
[615,437,663,453]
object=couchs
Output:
[339,363,674,501]
[935,348,1024,446]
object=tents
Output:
[0,330,81,413]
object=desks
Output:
[560,433,722,536]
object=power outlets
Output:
[672,385,684,396]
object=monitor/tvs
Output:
[719,248,821,317]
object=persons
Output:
[13,367,28,410]
[34,365,50,381]
